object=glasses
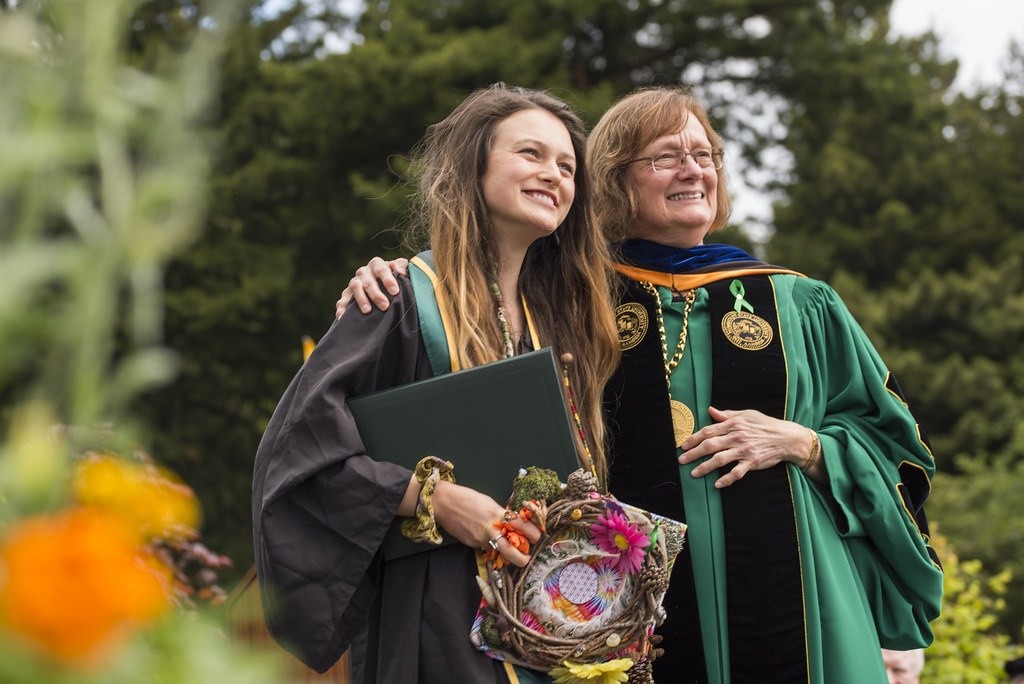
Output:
[624,148,725,174]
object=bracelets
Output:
[801,428,819,473]
[401,455,456,546]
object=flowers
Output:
[481,490,669,684]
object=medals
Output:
[669,400,695,449]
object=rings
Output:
[488,534,503,550]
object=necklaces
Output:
[477,252,600,492]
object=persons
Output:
[335,82,944,684]
[251,80,634,684]
